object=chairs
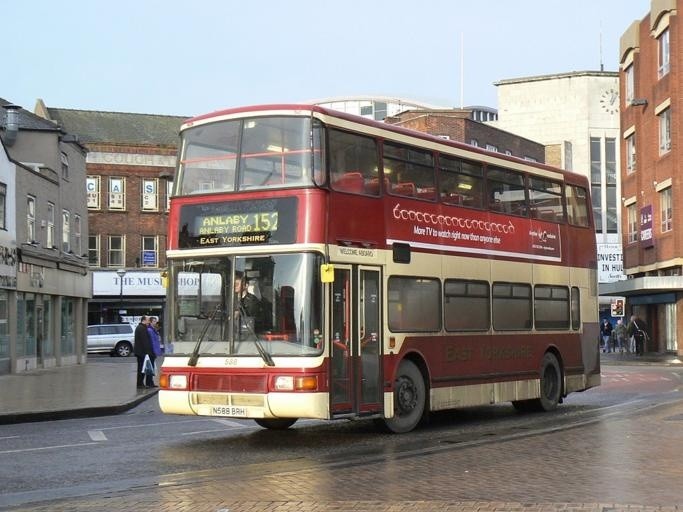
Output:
[335,173,587,227]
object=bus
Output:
[157,103,602,434]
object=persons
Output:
[225,276,264,333]
[600,315,647,356]
[134,316,160,389]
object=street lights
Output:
[116,269,127,323]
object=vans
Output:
[87,323,135,357]
